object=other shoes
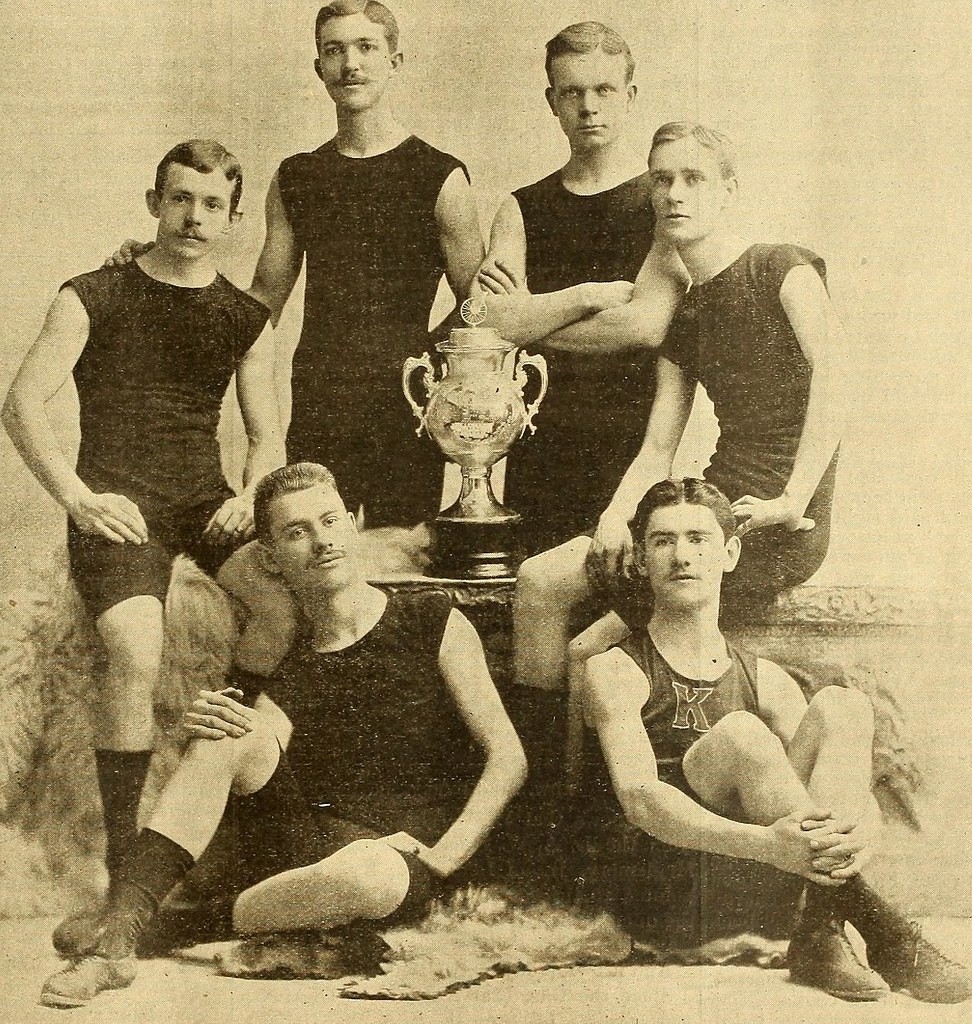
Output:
[866,921,972,1003]
[788,919,892,1000]
[40,948,138,1008]
[53,912,169,953]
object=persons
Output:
[102,1,485,527]
[1,141,302,955]
[493,123,858,840]
[476,22,694,555]
[40,460,531,1006]
[575,479,970,1004]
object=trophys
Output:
[399,300,547,576]
